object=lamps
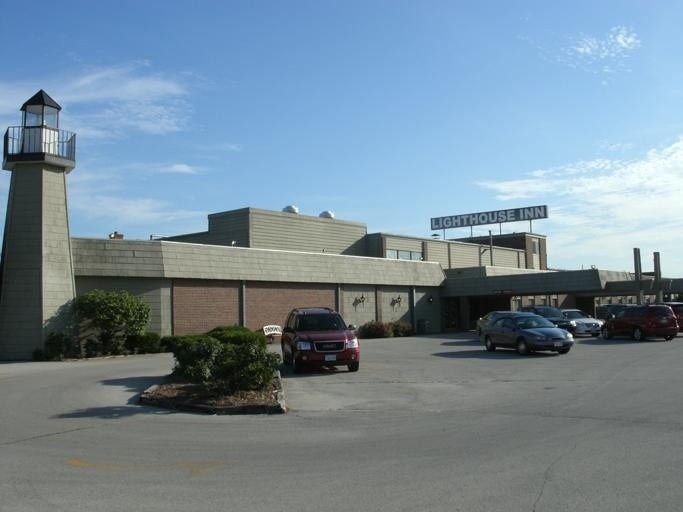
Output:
[360,296,433,307]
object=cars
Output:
[281,307,360,373]
[476,302,683,355]
